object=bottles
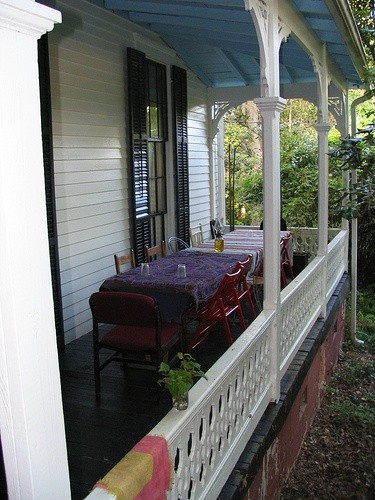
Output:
[214,234,225,252]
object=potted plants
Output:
[155,352,207,411]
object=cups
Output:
[140,263,150,277]
[177,264,186,278]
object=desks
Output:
[99,252,253,371]
[183,230,291,312]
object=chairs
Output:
[88,216,292,398]
